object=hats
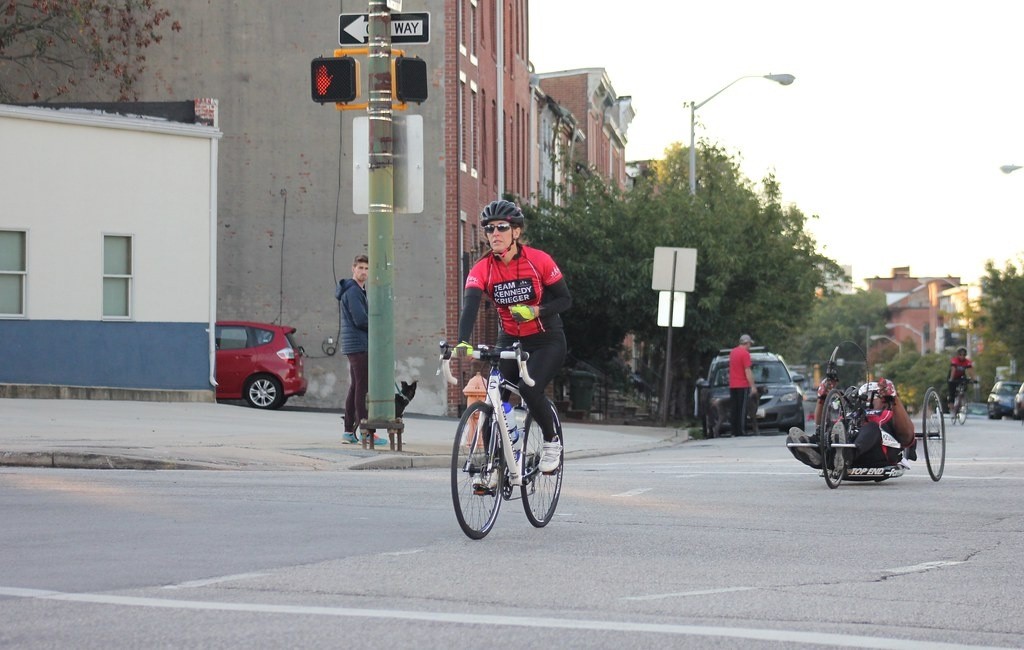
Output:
[739,334,754,345]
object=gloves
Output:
[817,377,837,401]
[877,377,897,399]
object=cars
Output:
[1014,383,1024,418]
[215,322,310,409]
[987,382,1022,420]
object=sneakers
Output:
[539,435,563,473]
[342,432,358,444]
[358,432,388,446]
[472,470,499,491]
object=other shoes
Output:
[788,427,822,466]
[831,421,855,468]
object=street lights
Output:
[871,335,901,358]
[688,71,796,189]
[919,277,971,357]
[885,322,926,356]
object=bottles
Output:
[502,402,523,451]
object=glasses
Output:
[482,223,516,233]
[960,353,966,356]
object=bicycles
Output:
[436,341,565,539]
[951,379,972,425]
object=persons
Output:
[787,378,915,470]
[453,201,573,492]
[947,349,976,414]
[335,254,388,446]
[729,333,758,437]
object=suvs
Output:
[695,346,805,436]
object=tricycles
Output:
[788,342,947,490]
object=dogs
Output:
[340,380,418,444]
[712,384,768,439]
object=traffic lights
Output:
[393,57,428,103]
[310,57,361,102]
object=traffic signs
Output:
[340,11,429,45]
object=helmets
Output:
[858,381,887,417]
[958,347,966,355]
[480,200,524,228]
